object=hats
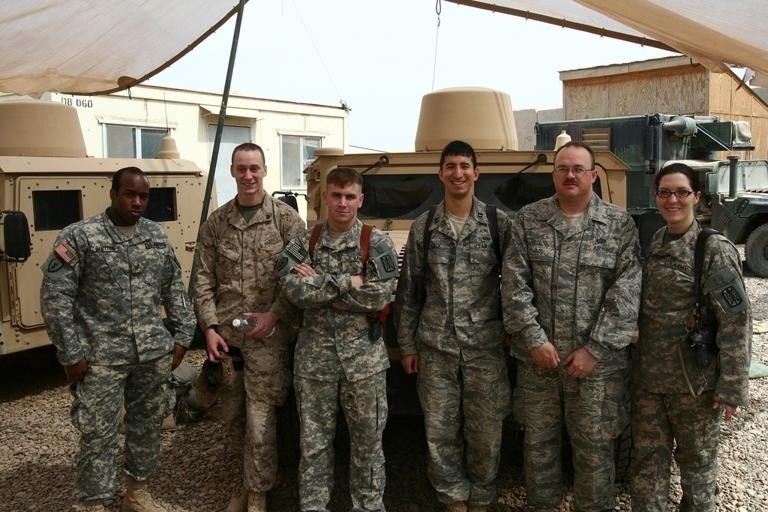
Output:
[196,353,235,409]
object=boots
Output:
[83,499,109,512]
[122,479,165,511]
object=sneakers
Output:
[228,490,266,512]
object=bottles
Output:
[231,315,281,339]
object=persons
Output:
[191,142,310,511]
[499,142,643,510]
[40,165,199,511]
[628,161,753,512]
[275,168,400,511]
[392,138,513,512]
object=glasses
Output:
[554,166,593,177]
[654,188,695,200]
